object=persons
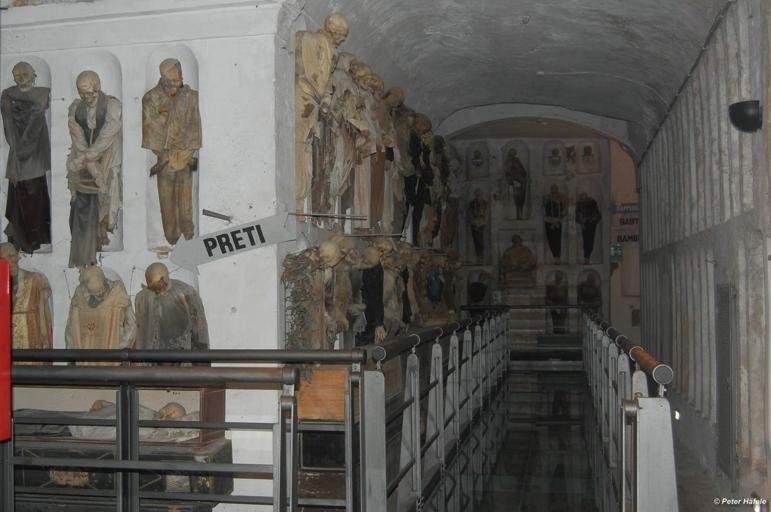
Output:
[0,11,602,443]
